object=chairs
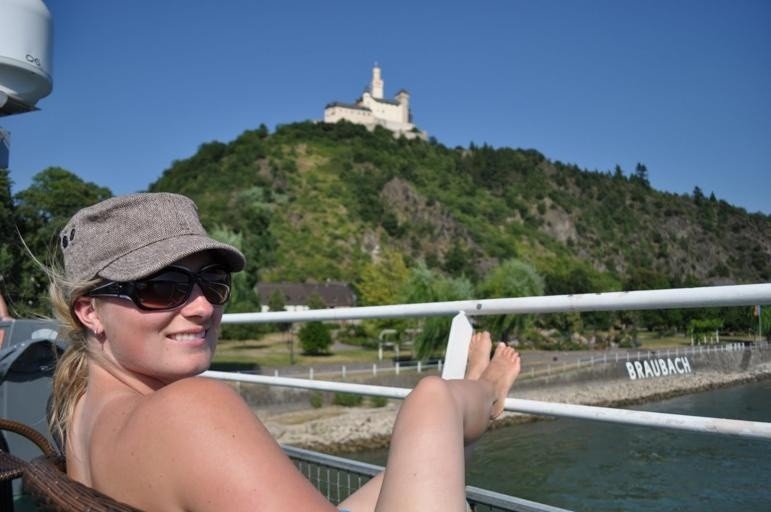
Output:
[0,417,141,512]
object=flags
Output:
[753,304,761,317]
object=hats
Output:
[60,190,246,290]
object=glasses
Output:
[81,263,233,311]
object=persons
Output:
[45,191,524,512]
[0,293,10,352]
